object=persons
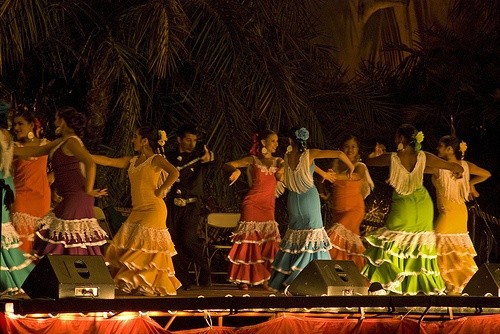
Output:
[163,126,220,291]
[358,123,464,296]
[222,127,285,291]
[89,125,180,296]
[267,126,355,294]
[324,130,376,296]
[0,106,110,297]
[425,134,491,295]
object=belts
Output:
[167,197,197,207]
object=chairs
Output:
[94,206,113,253]
[197,213,242,288]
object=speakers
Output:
[20,254,118,299]
[285,259,371,297]
[461,263,500,297]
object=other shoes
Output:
[241,283,249,291]
[199,274,208,287]
[263,282,277,292]
[184,280,193,291]
